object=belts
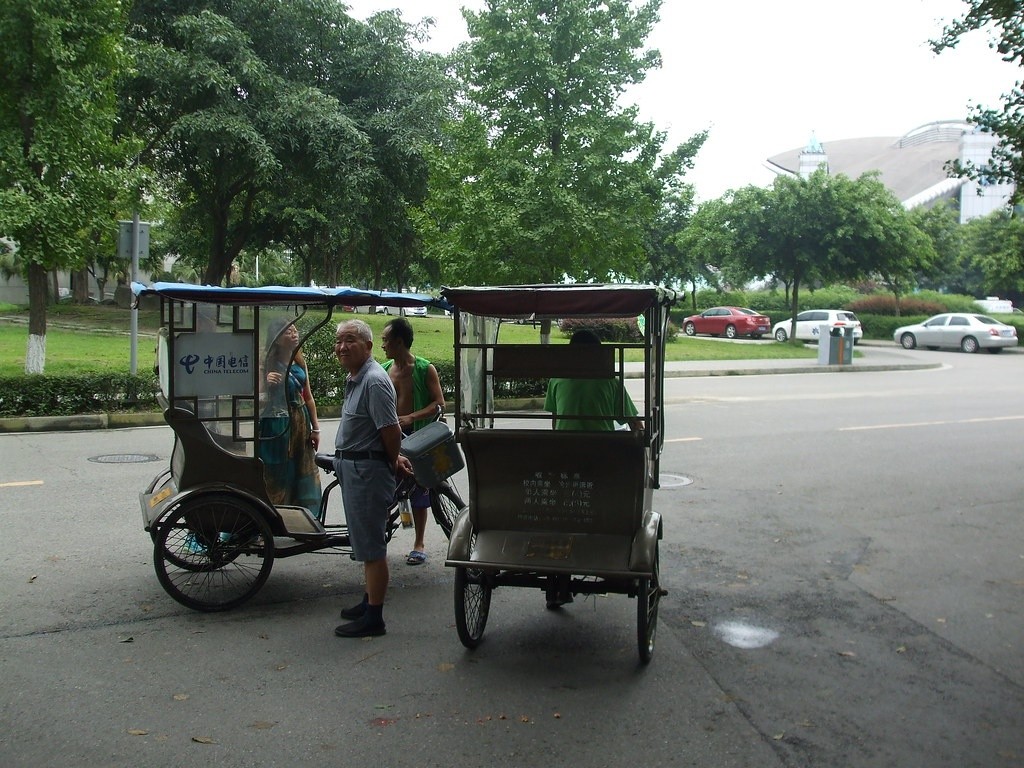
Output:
[335,450,389,461]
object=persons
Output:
[545,330,643,432]
[335,320,414,637]
[259,316,319,548]
[380,318,446,565]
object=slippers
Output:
[406,551,427,565]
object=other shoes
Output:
[546,590,574,609]
[333,604,386,638]
[340,591,369,621]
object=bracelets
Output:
[311,429,319,432]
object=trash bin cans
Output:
[817,324,855,366]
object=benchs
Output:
[460,431,658,566]
[157,392,272,511]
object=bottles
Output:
[398,492,414,530]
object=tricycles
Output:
[128,279,472,615]
[439,279,677,668]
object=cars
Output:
[353,306,385,314]
[336,303,353,312]
[59,296,99,304]
[893,312,1019,353]
[771,309,863,345]
[682,305,771,340]
[383,306,428,317]
